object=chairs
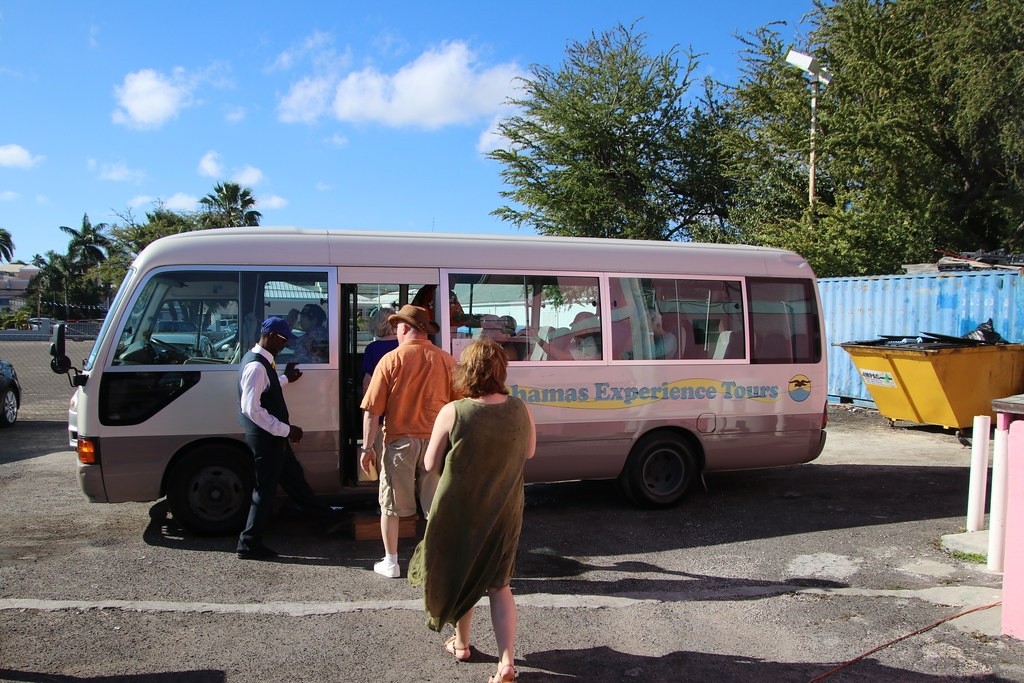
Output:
[529,326,554,362]
[674,320,810,362]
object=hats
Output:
[298,303,327,322]
[260,317,298,340]
[388,304,441,335]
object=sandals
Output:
[488,663,518,683]
[444,635,471,661]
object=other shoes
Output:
[237,547,280,560]
[322,506,355,534]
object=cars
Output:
[30,321,42,330]
[210,333,238,359]
[0,359,22,426]
[151,318,211,352]
[228,322,238,333]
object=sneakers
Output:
[373,557,401,578]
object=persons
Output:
[412,275,481,339]
[482,314,519,360]
[283,304,327,364]
[360,304,469,577]
[635,310,684,358]
[362,309,399,395]
[529,311,598,360]
[235,317,355,560]
[407,338,536,683]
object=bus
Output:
[47,228,830,540]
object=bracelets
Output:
[361,446,373,453]
[538,339,544,347]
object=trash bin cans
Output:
[830,336,1024,434]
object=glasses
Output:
[276,333,289,346]
